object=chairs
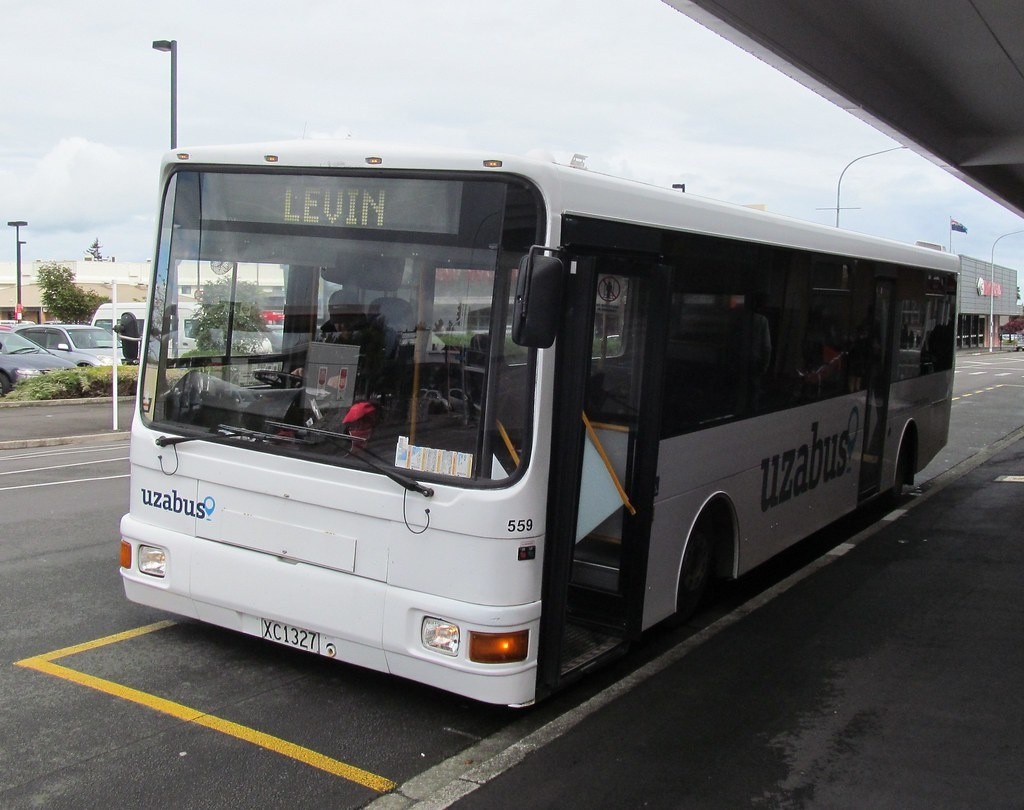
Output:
[369,293,417,359]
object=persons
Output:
[291,290,396,405]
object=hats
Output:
[341,401,377,424]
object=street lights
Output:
[835,141,924,230]
[990,231,1023,354]
[152,38,178,152]
[10,218,29,303]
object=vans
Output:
[256,309,283,351]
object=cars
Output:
[1,324,124,397]
[0,329,78,397]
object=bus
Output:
[119,147,961,709]
[92,302,273,366]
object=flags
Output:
[951,218,968,234]
